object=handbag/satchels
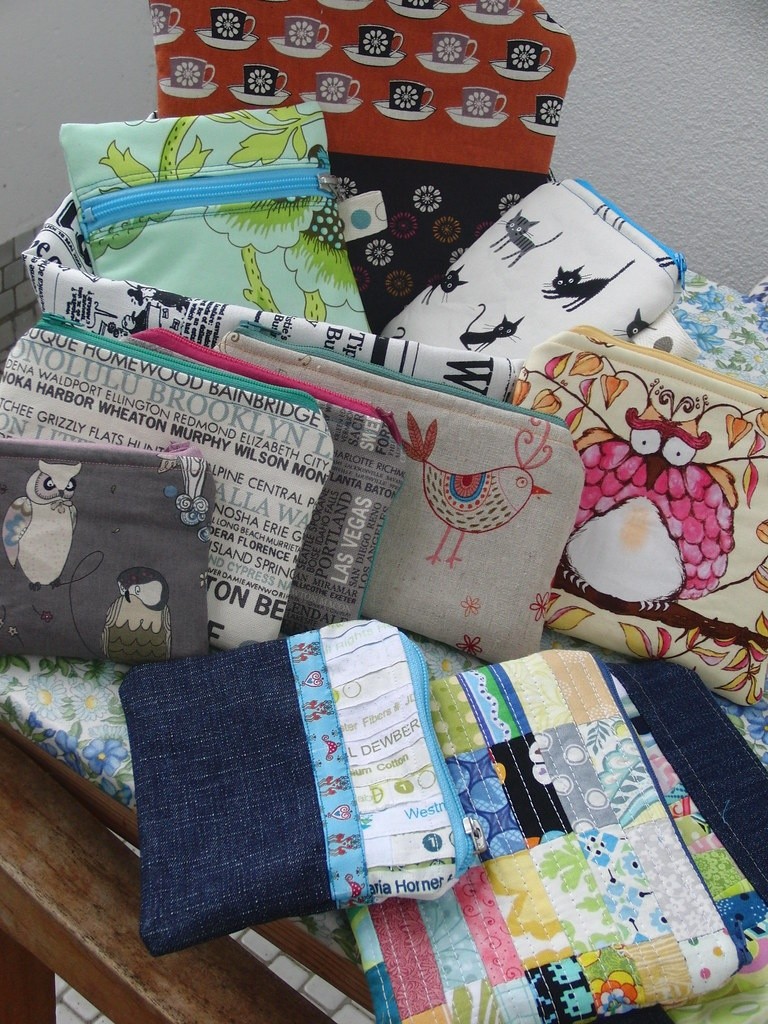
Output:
[119,619,488,957]
[209,327,586,662]
[0,439,217,660]
[149,0,577,314]
[0,311,334,650]
[512,323,768,707]
[114,326,405,636]
[343,648,768,1024]
[382,178,687,362]
[58,102,368,332]
